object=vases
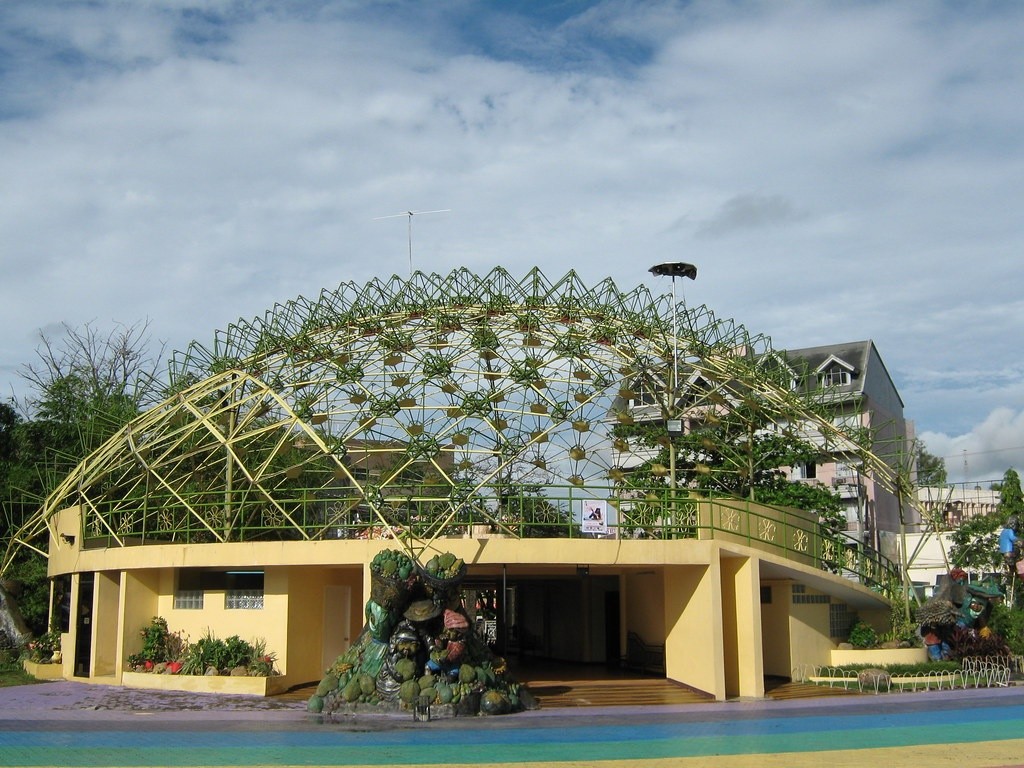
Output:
[51,650,62,664]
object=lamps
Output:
[576,564,591,577]
[665,419,685,437]
